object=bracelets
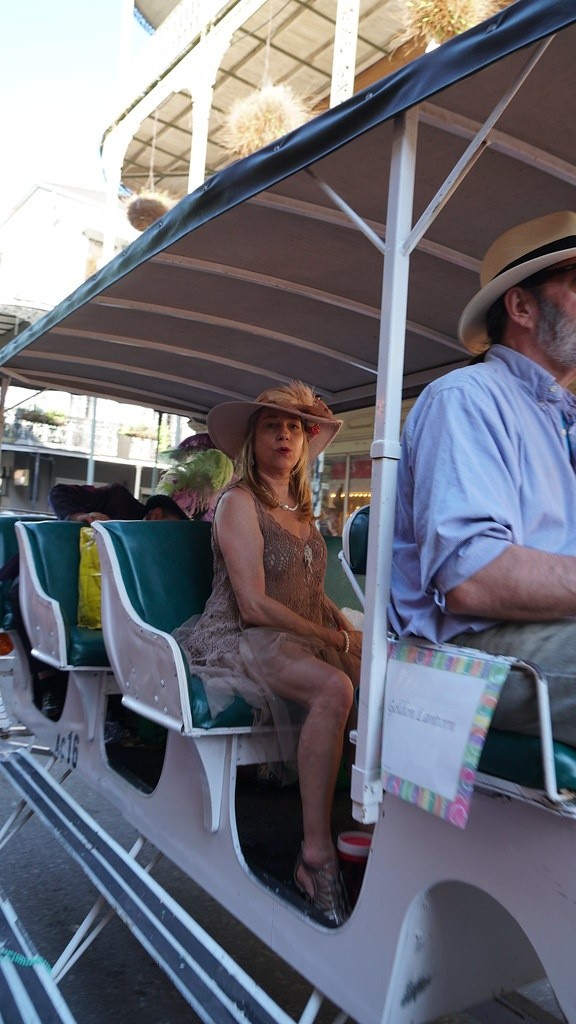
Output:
[342,630,349,653]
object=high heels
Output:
[295,841,352,928]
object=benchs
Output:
[0,510,59,672]
[13,520,114,671]
[90,518,365,738]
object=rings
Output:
[90,513,95,517]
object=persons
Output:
[48,482,190,524]
[173,381,362,928]
[384,210,575,747]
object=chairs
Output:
[335,504,576,817]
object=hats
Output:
[145,449,234,520]
[457,210,576,354]
[205,379,343,463]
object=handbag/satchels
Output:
[77,525,102,630]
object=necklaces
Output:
[263,487,299,511]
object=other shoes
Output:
[40,677,62,718]
